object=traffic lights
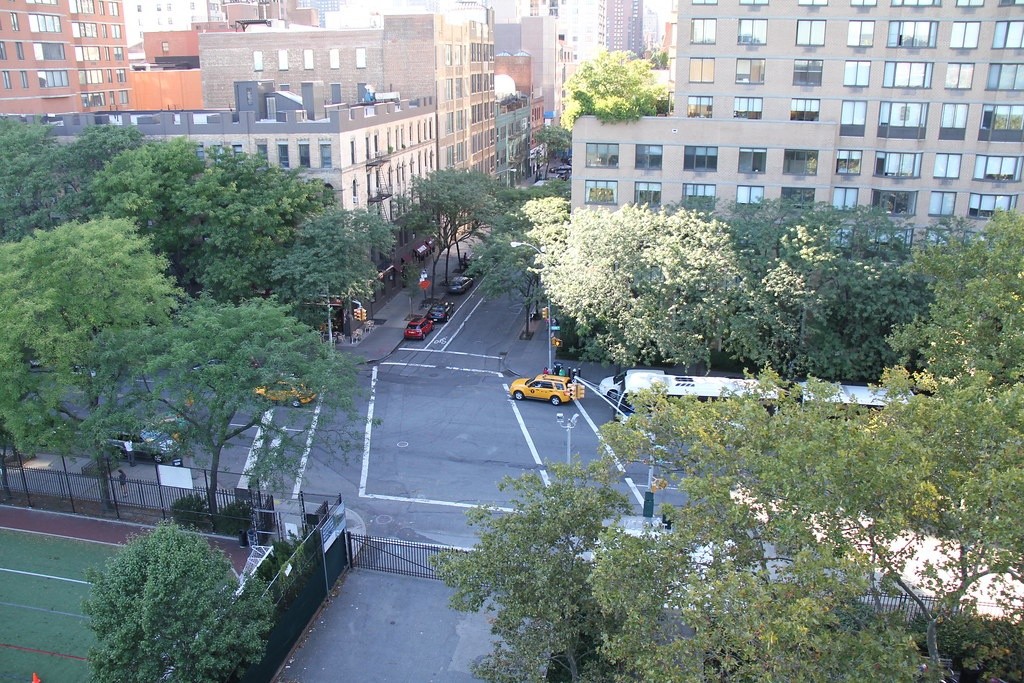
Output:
[541,307,548,320]
[362,309,367,320]
[357,308,361,321]
[569,387,575,401]
[555,340,561,347]
[567,384,572,397]
[578,384,585,400]
[353,309,357,319]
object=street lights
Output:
[510,241,553,375]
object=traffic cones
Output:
[31,672,41,683]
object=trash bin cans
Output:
[662,514,672,530]
[247,528,261,548]
[238,530,246,547]
[554,361,563,375]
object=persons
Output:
[117,469,128,498]
[559,367,565,377]
[543,366,549,374]
[124,439,137,467]
[463,252,467,265]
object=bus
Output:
[794,381,915,422]
[612,374,779,431]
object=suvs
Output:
[509,374,571,406]
[447,277,474,295]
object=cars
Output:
[549,165,572,173]
[192,358,227,374]
[252,380,318,407]
[599,370,665,400]
[404,317,435,341]
[426,300,455,322]
[105,428,180,465]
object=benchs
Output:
[923,656,958,683]
